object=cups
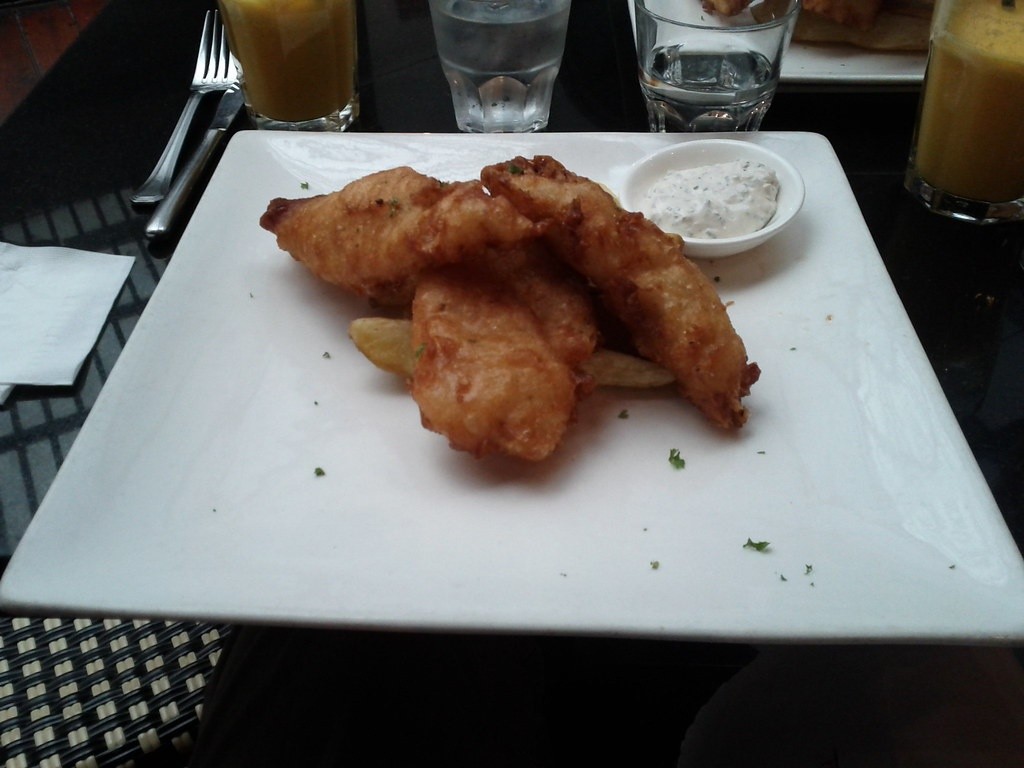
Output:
[218,0,360,132]
[635,0,801,133]
[430,0,572,133]
[903,0,1024,226]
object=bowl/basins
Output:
[619,138,806,259]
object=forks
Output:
[129,10,237,204]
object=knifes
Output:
[142,77,244,240]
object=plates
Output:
[0,130,1024,645]
[628,0,924,93]
